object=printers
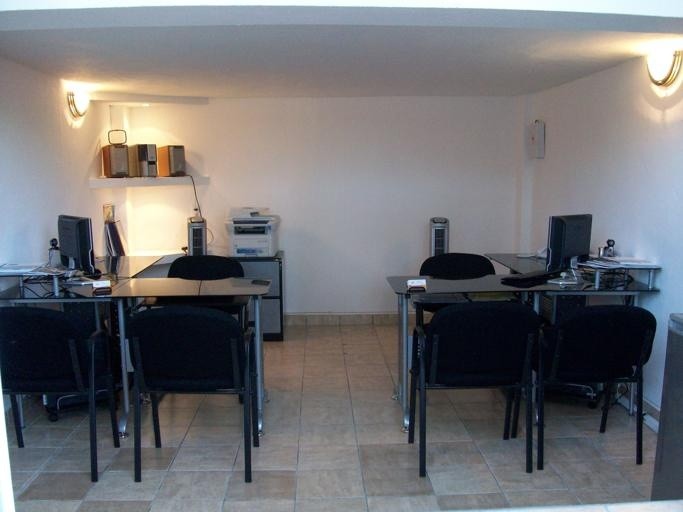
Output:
[225,206,280,258]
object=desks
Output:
[1,257,273,436]
[387,253,662,434]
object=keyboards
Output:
[501,269,567,288]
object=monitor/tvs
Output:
[547,214,592,285]
[57,215,102,280]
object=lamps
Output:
[67,92,90,118]
[646,50,683,87]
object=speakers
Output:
[102,145,130,178]
[158,146,186,176]
[130,144,157,177]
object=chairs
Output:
[409,302,537,472]
[511,305,656,464]
[155,255,249,404]
[126,305,259,483]
[414,252,495,326]
[1,306,120,481]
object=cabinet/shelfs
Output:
[237,251,285,342]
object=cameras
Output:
[598,239,615,257]
